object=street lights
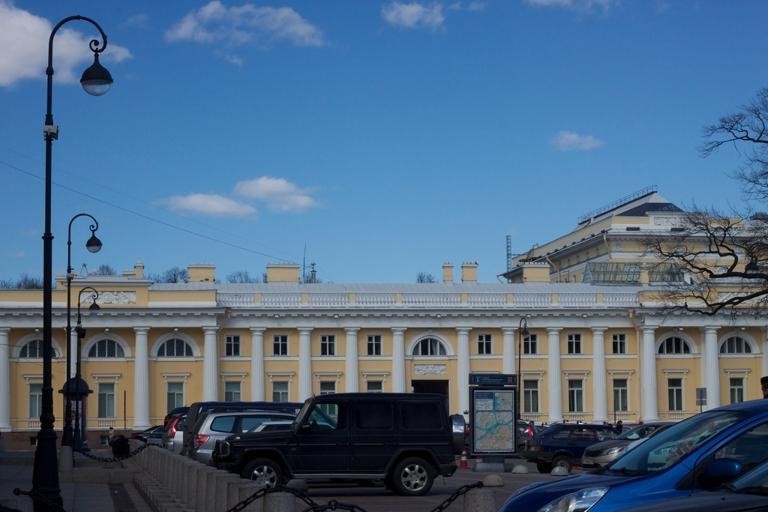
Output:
[64,214,102,425]
[40,16,113,431]
[74,286,101,425]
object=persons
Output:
[108,427,114,439]
[616,421,623,433]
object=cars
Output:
[497,398,767,511]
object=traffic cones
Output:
[459,448,469,469]
